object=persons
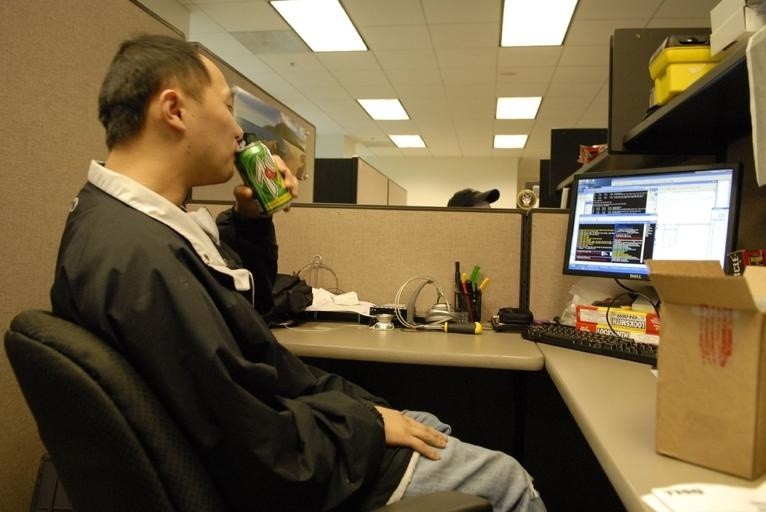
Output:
[44,29,553,512]
[447,187,502,209]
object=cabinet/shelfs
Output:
[541,26,766,209]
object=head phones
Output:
[393,273,465,329]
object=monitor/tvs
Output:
[562,160,745,282]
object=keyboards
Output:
[520,321,658,367]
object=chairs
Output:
[3,308,212,511]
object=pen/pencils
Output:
[455,261,489,322]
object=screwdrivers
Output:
[415,321,482,335]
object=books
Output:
[724,245,766,278]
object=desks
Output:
[536,334,762,510]
[268,314,544,372]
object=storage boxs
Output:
[646,258,765,480]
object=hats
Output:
[448,189,499,207]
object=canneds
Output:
[234,141,293,218]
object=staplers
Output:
[369,307,407,328]
